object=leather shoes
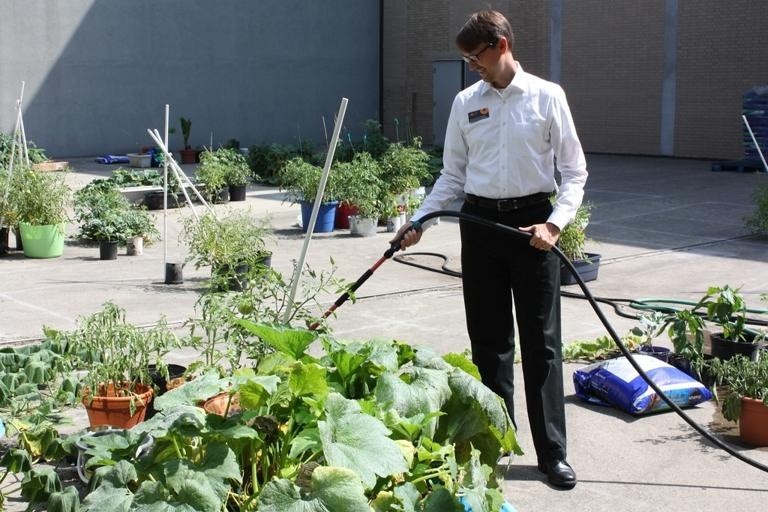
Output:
[537,458,576,489]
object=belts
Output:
[466,193,549,212]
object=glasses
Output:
[460,42,495,65]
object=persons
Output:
[388,12,590,490]
